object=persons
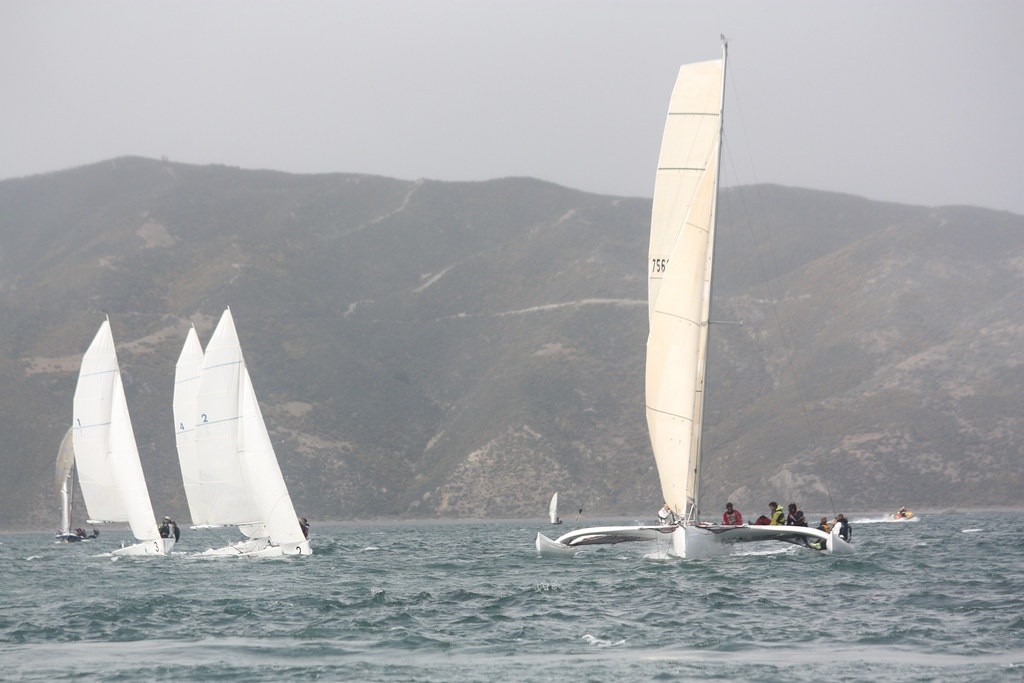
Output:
[809,511,853,551]
[299,517,310,538]
[155,515,182,541]
[786,503,807,527]
[720,502,743,525]
[900,506,907,517]
[657,503,671,525]
[747,502,785,525]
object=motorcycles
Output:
[893,512,914,521]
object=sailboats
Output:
[68,312,178,561]
[549,492,563,525]
[171,305,315,562]
[53,423,98,542]
[536,35,852,560]
